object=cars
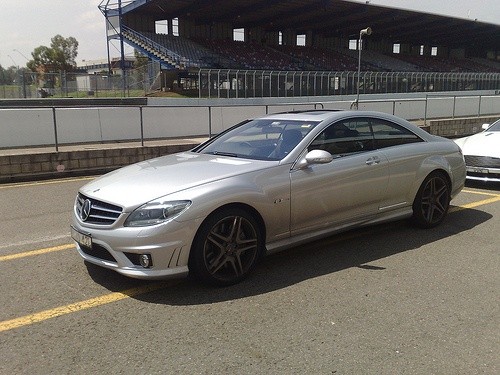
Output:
[70,109,468,289]
[454,117,500,189]
